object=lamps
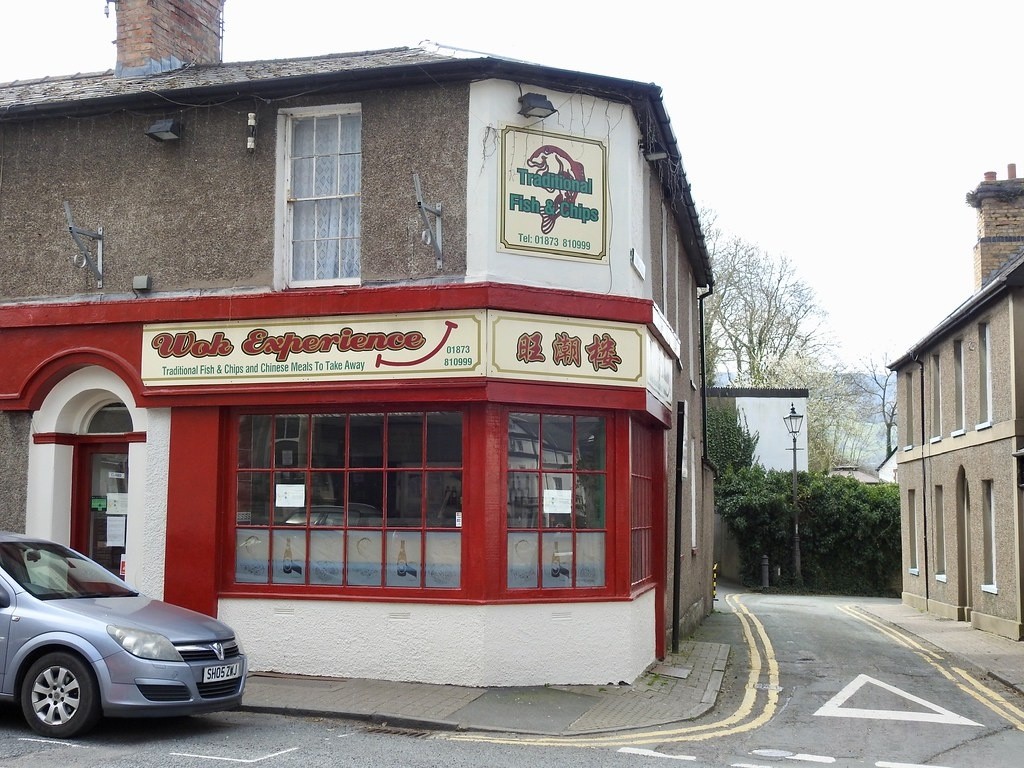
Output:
[145,118,183,143]
[516,92,561,121]
[638,138,668,161]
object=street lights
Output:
[783,402,804,593]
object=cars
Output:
[0,532,248,739]
[285,498,382,527]
